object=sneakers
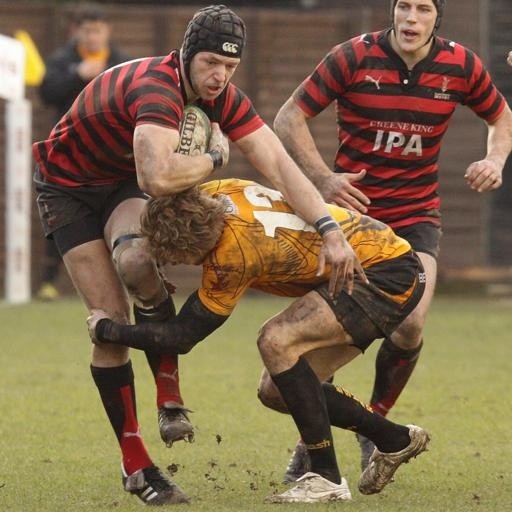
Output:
[158,401,197,446]
[354,422,429,496]
[121,460,191,507]
[285,441,311,482]
[263,471,352,505]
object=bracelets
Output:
[207,147,225,174]
[310,213,341,237]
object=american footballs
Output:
[175,104,212,155]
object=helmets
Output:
[182,4,245,93]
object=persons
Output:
[34,4,139,304]
[86,176,434,508]
[28,3,374,508]
[253,1,512,493]
